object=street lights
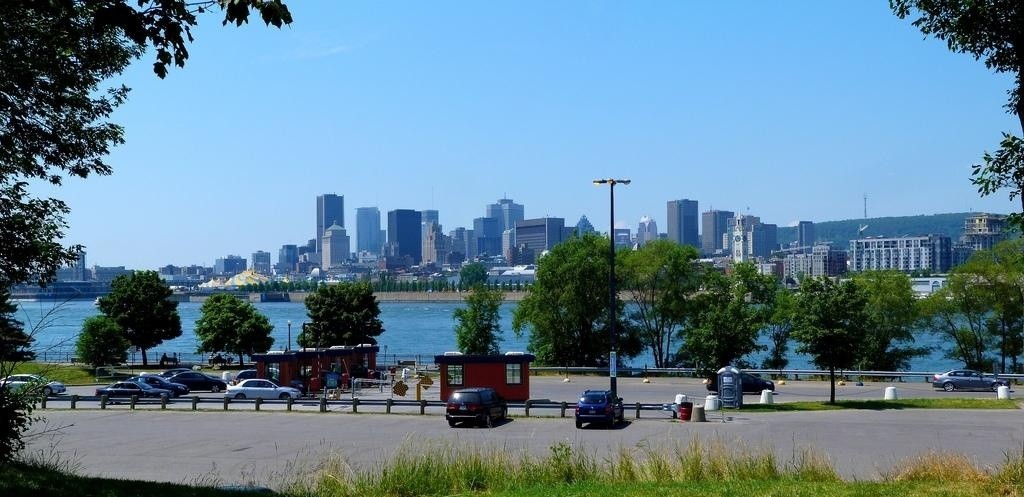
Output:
[593,178,631,395]
[287,320,291,350]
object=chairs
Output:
[257,382,262,387]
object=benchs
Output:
[163,358,177,367]
[208,358,234,366]
[71,358,84,366]
[397,360,416,368]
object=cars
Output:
[96,368,303,405]
[932,369,1010,393]
[575,390,624,428]
[706,370,774,394]
[446,387,507,427]
[0,374,66,396]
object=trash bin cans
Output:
[680,402,693,420]
